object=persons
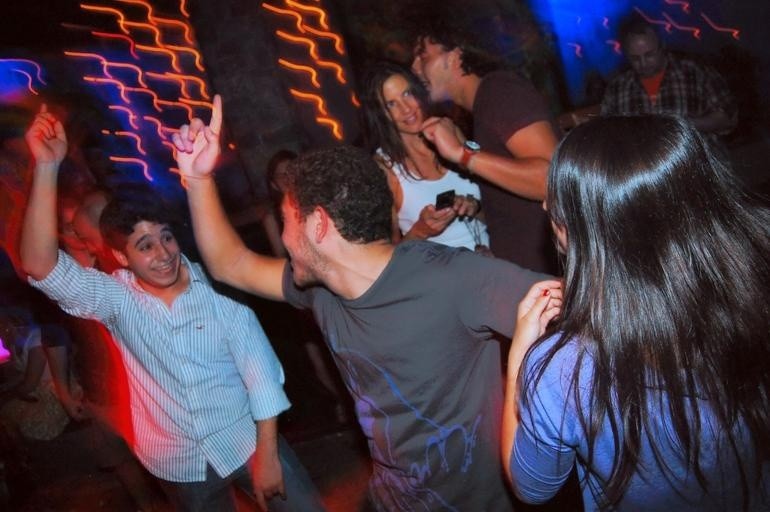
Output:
[1,0,770,512]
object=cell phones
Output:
[436,190,455,211]
